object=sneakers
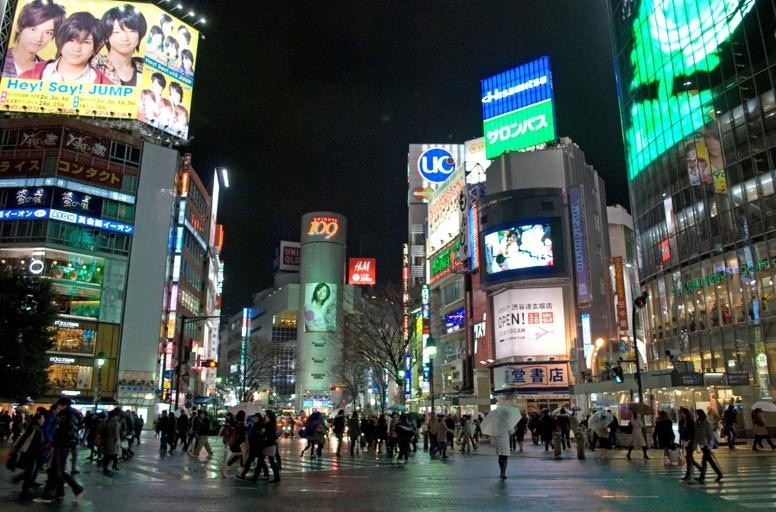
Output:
[302,447,476,464]
[71,488,86,502]
[237,474,282,485]
[33,496,52,502]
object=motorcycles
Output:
[281,420,296,438]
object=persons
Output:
[221,410,282,483]
[422,412,484,459]
[2,1,193,139]
[581,409,618,461]
[685,121,727,194]
[626,407,723,483]
[510,407,586,456]
[305,281,337,333]
[2,396,85,505]
[750,409,775,450]
[155,410,213,456]
[706,404,737,451]
[83,407,144,474]
[495,427,515,480]
[284,409,421,463]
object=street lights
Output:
[366,385,372,407]
[92,352,107,414]
[398,364,407,395]
[424,334,438,415]
[216,373,222,411]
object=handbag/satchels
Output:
[300,429,308,437]
[669,448,681,462]
[752,415,764,426]
[276,428,283,439]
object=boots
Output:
[238,467,244,474]
[219,464,231,479]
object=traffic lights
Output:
[634,297,646,309]
[612,367,624,384]
[183,347,190,361]
[331,386,340,391]
[205,360,218,368]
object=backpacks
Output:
[225,422,241,444]
